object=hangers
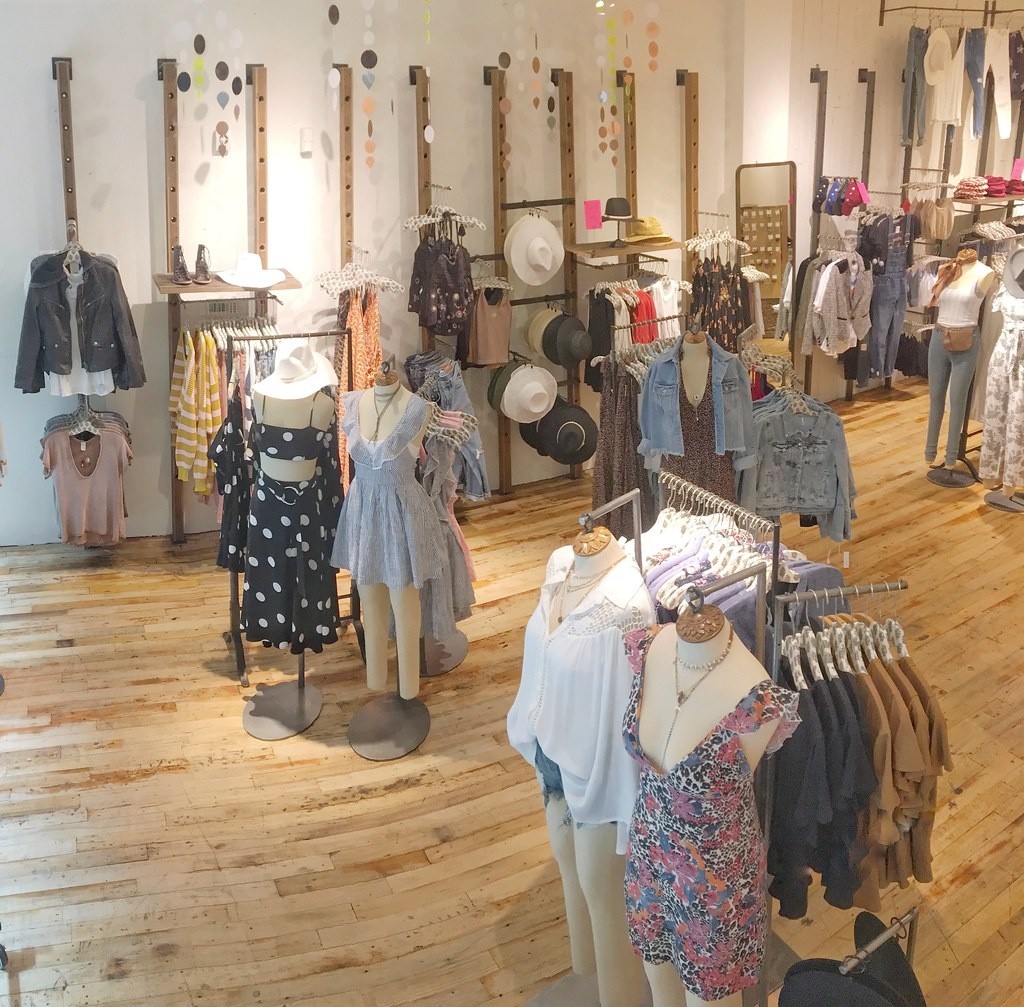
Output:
[618,472,940,757]
[29,220,118,279]
[472,258,514,293]
[402,181,489,232]
[315,243,407,301]
[45,391,127,440]
[586,160,957,427]
[179,316,281,354]
[958,203,1024,273]
[413,375,479,452]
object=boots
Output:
[194,244,211,284]
[172,245,192,284]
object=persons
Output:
[637,330,759,527]
[925,247,995,472]
[331,370,447,699]
[503,528,651,1007]
[621,605,799,1007]
[237,343,342,653]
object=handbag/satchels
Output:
[935,324,975,352]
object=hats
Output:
[503,214,566,287]
[215,253,286,288]
[523,306,592,369]
[812,176,863,216]
[253,338,339,399]
[621,217,672,243]
[519,397,599,465]
[600,198,646,223]
[923,28,951,86]
[486,358,559,424]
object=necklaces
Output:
[553,555,625,622]
[662,634,724,765]
[368,382,400,444]
[674,626,731,669]
